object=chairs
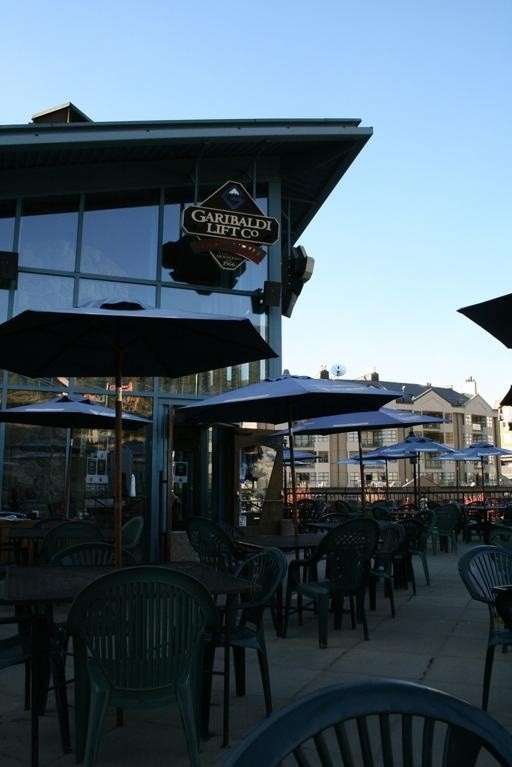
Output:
[434,496,512,552]
[187,498,434,617]
[458,545,512,711]
[0,516,145,568]
[285,519,380,650]
[226,677,512,767]
[0,617,44,765]
[67,567,217,766]
[217,547,288,749]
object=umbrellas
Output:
[0,393,152,522]
[0,299,278,567]
